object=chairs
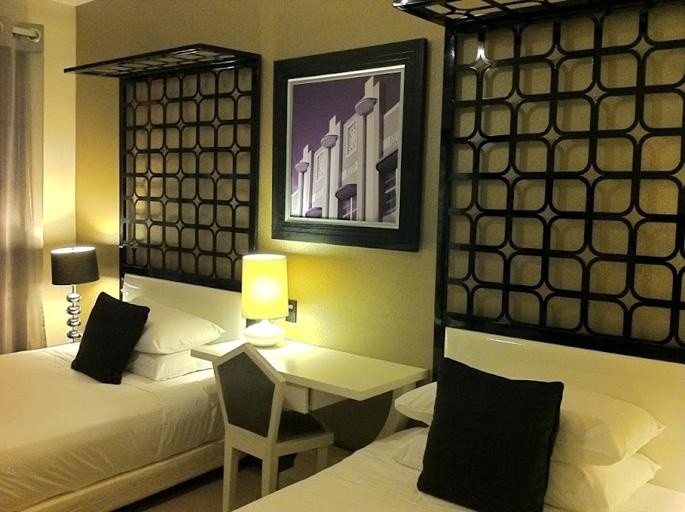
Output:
[212,343,335,512]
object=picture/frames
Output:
[270,38,427,252]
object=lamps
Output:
[51,247,100,345]
[241,255,290,347]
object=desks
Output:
[190,334,430,482]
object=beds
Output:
[0,273,250,512]
[231,327,685,512]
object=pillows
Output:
[71,292,150,384]
[394,366,666,467]
[127,345,225,381]
[126,295,226,355]
[416,357,564,512]
[394,427,662,512]
